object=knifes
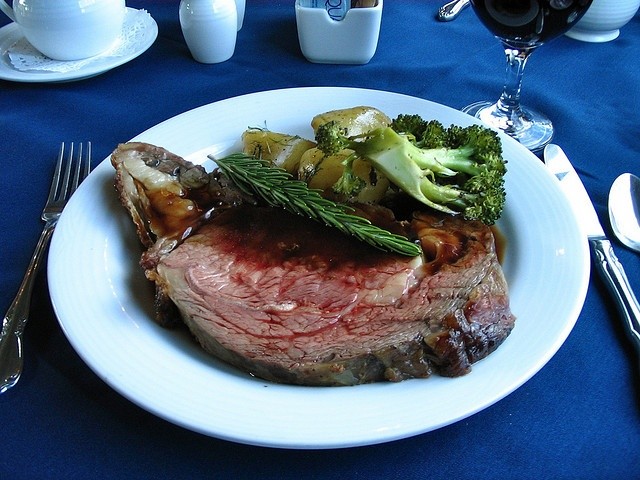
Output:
[542,142,640,353]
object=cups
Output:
[12,0,124,61]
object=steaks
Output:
[110,142,515,385]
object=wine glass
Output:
[451,0,594,151]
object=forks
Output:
[0,139,93,400]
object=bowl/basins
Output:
[562,0,640,44]
[294,1,385,64]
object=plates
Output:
[48,84,593,451]
[5,6,159,83]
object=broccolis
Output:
[361,110,511,231]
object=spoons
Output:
[607,173,640,252]
